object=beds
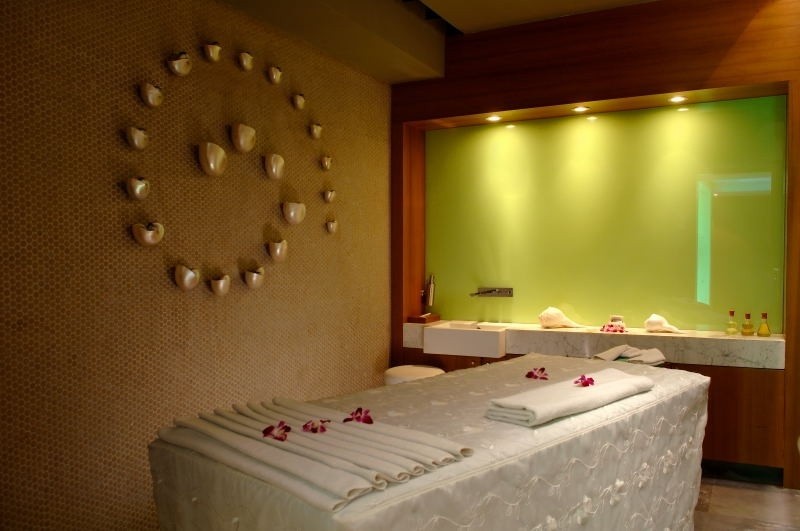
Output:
[148,352,710,531]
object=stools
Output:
[385,363,446,385]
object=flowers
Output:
[574,374,594,387]
[528,366,549,380]
[262,407,373,441]
[602,323,625,333]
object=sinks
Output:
[424,321,511,358]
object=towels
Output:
[487,368,655,427]
[592,344,666,366]
[156,393,476,513]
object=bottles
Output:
[741,313,754,336]
[757,313,771,337]
[725,310,738,335]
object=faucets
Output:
[469,289,499,298]
